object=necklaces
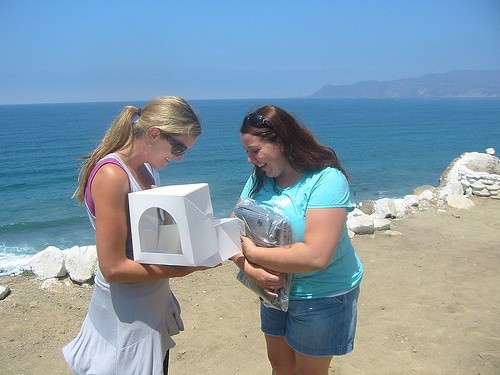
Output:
[274,168,296,196]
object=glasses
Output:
[244,113,274,130]
[165,134,188,156]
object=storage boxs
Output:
[127,182,242,268]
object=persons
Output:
[227,105,363,375]
[61,94,221,375]
